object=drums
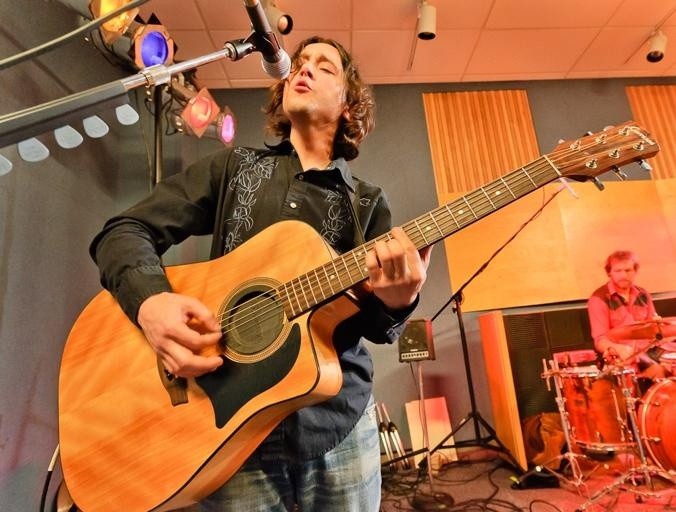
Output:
[639,378,675,483]
[658,352,676,380]
[561,364,640,452]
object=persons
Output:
[587,250,676,439]
[88,34,436,510]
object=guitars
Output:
[58,120,660,507]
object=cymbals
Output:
[595,341,655,381]
[609,317,676,339]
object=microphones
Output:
[243,0,291,79]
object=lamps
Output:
[259,0,292,34]
[63,0,236,147]
[646,26,668,64]
[414,0,439,42]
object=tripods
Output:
[418,296,529,474]
[536,358,611,503]
[577,336,676,512]
